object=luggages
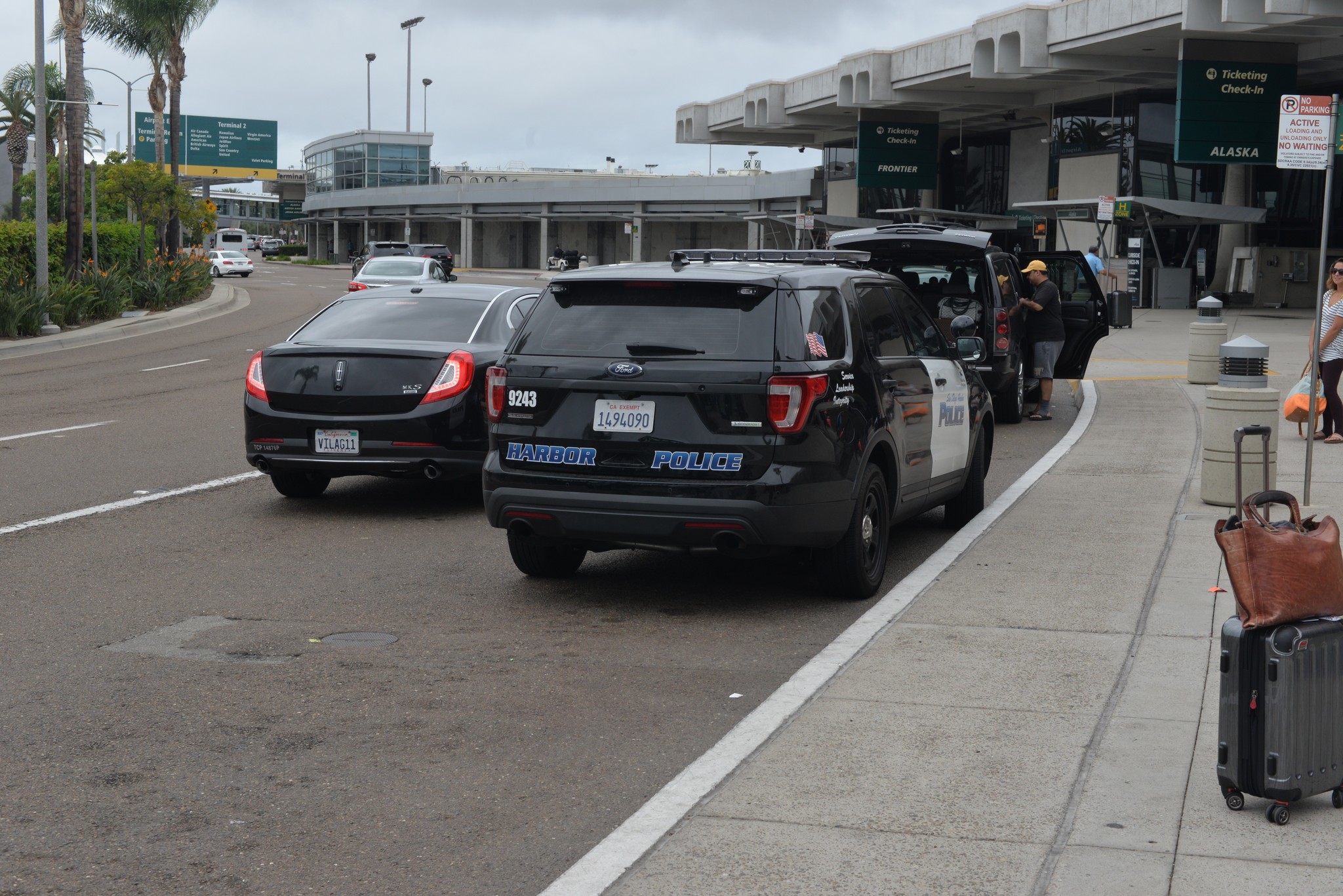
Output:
[1216,425,1343,826]
[1106,276,1132,329]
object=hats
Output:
[997,275,1009,286]
[1021,260,1047,273]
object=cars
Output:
[210,237,214,249]
[202,250,254,278]
[241,283,539,500]
[246,234,285,257]
[347,255,458,295]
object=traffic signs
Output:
[135,111,278,180]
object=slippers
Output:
[1314,432,1326,440]
[1323,434,1343,444]
[1023,411,1034,417]
[1028,413,1052,420]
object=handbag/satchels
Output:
[1284,358,1327,440]
[937,296,984,323]
[1214,490,1343,630]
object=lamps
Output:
[799,145,805,153]
[950,111,962,155]
[1041,89,1055,143]
[844,131,855,167]
[1099,83,1115,136]
[1003,109,1016,120]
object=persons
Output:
[998,275,1022,317]
[283,236,353,262]
[1073,245,1117,294]
[1019,260,1065,421]
[1308,258,1343,443]
[554,245,563,258]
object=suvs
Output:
[410,243,453,277]
[348,240,415,281]
[480,246,995,600]
[823,219,1112,424]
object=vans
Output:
[213,228,248,258]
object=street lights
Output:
[422,79,433,132]
[365,53,376,131]
[83,65,188,268]
[400,17,425,131]
[748,151,759,160]
[645,164,658,173]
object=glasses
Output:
[1329,268,1343,276]
